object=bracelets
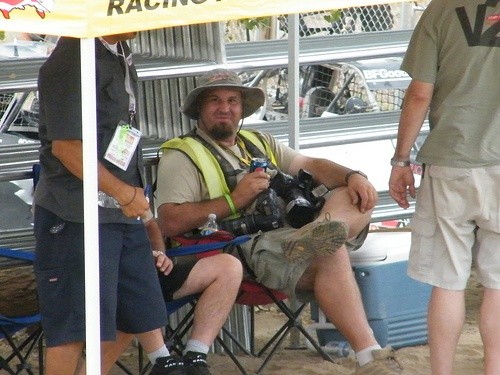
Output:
[346,170,368,183]
[158,250,165,254]
[118,183,136,207]
[224,193,236,214]
[390,158,410,167]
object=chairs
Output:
[304,86,336,117]
[0,156,335,375]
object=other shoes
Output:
[152,356,184,375]
[179,351,212,375]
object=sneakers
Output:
[281,212,348,264]
[353,346,405,375]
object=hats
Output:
[183,68,265,120]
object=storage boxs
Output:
[310,231,433,351]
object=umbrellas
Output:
[0,0,375,375]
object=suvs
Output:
[1,62,403,147]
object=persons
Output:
[385,0,500,375]
[34,31,169,375]
[156,70,412,375]
[134,216,243,375]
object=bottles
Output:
[202,213,218,236]
[325,341,356,358]
